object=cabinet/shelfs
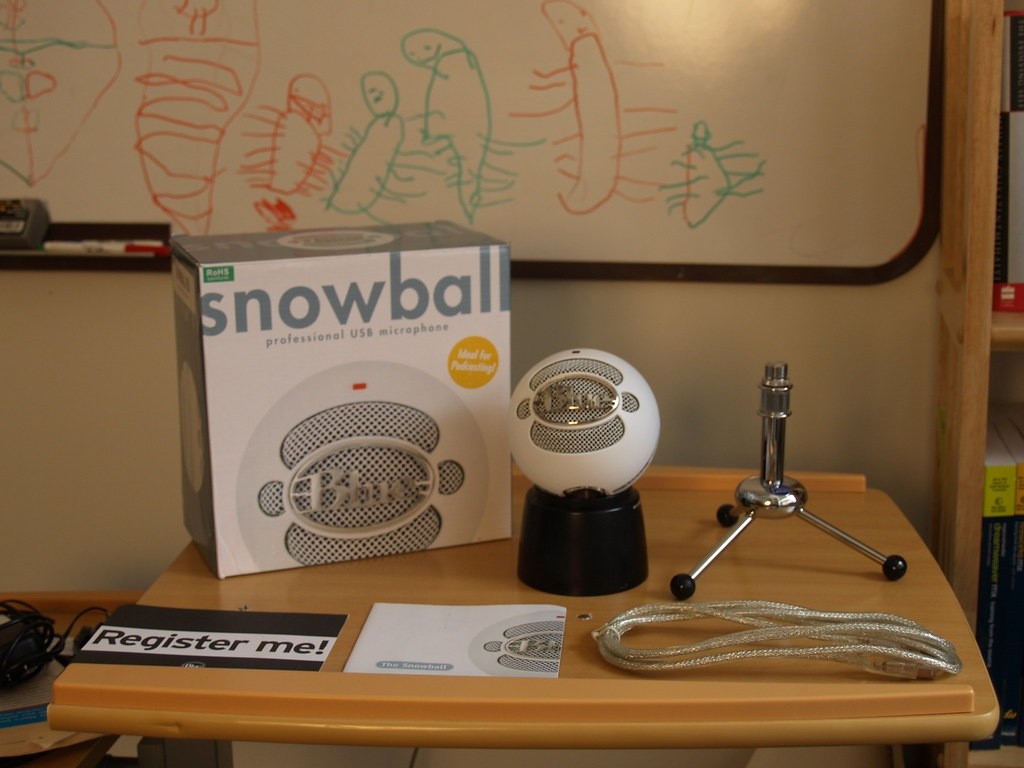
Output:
[936,0,1024,768]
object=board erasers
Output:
[0,197,49,250]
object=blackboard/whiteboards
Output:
[0,1,949,289]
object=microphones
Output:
[44,606,111,662]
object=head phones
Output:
[0,598,55,694]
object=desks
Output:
[47,466,1001,768]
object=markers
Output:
[37,238,173,258]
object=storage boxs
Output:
[169,220,512,580]
